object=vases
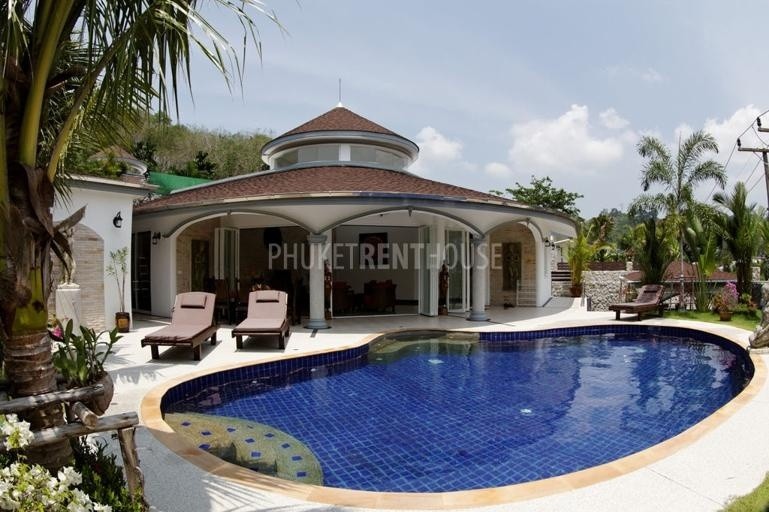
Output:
[719,310,734,319]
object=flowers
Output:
[0,413,111,512]
[719,281,739,310]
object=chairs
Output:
[231,288,288,350]
[140,290,218,360]
[609,284,666,321]
[208,273,398,314]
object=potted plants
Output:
[50,317,123,415]
[106,248,133,333]
[565,221,608,298]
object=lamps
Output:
[151,232,161,246]
[113,211,123,229]
[543,237,551,248]
